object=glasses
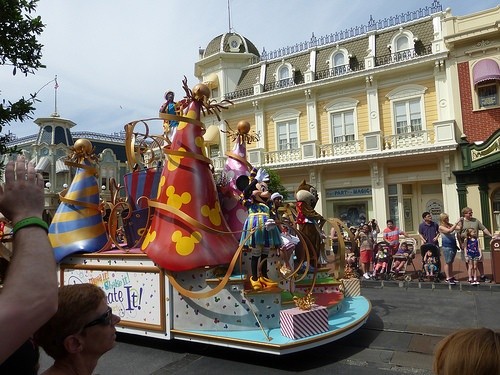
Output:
[464,211,473,214]
[85,305,113,326]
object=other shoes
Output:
[445,276,459,285]
[480,275,491,283]
[363,272,371,280]
[467,279,480,285]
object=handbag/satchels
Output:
[438,234,442,247]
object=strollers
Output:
[418,241,443,283]
[369,241,393,281]
[387,237,420,282]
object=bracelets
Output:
[12,218,49,234]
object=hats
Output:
[349,226,357,233]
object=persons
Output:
[0,151,57,375]
[35,283,120,375]
[432,327,500,375]
[159,90,183,153]
[417,206,488,289]
[332,219,415,280]
